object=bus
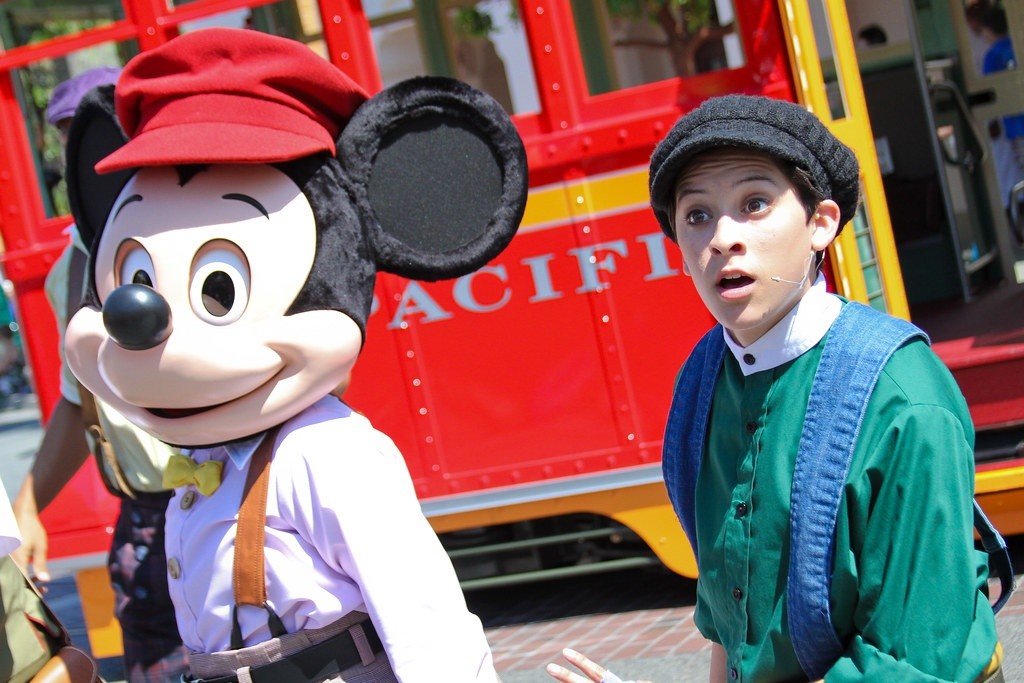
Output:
[0,0,1024,590]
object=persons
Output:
[549,95,1004,683]
[0,69,183,683]
[969,0,1023,244]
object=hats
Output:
[43,65,126,125]
[646,93,860,243]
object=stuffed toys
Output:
[0,28,530,683]
